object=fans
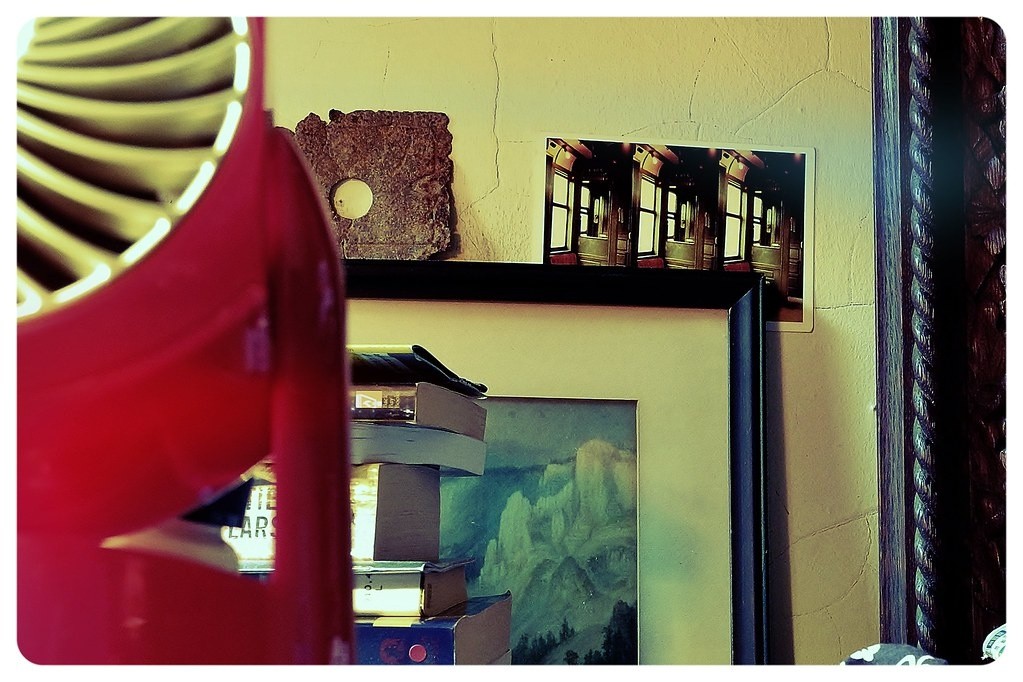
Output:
[17,16,359,668]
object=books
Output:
[223,340,518,665]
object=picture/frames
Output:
[343,259,771,665]
